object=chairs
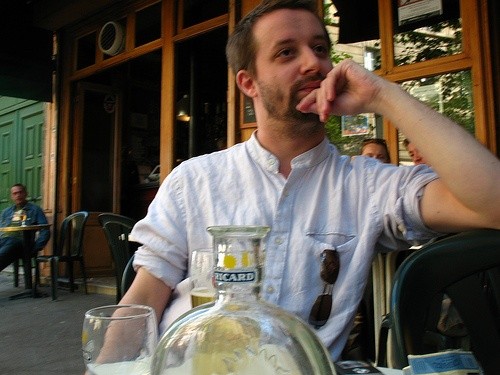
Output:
[12,253,41,287]
[33,209,88,300]
[98,211,142,305]
[376,230,500,375]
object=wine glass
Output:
[19,210,28,227]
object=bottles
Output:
[150,226,336,375]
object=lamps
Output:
[177,110,191,122]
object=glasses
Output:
[363,138,387,144]
[308,248,340,331]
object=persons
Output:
[402,138,425,165]
[359,139,390,163]
[0,183,51,273]
[86,0,500,375]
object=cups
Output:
[82,304,157,375]
[189,248,218,308]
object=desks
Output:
[0,223,54,301]
